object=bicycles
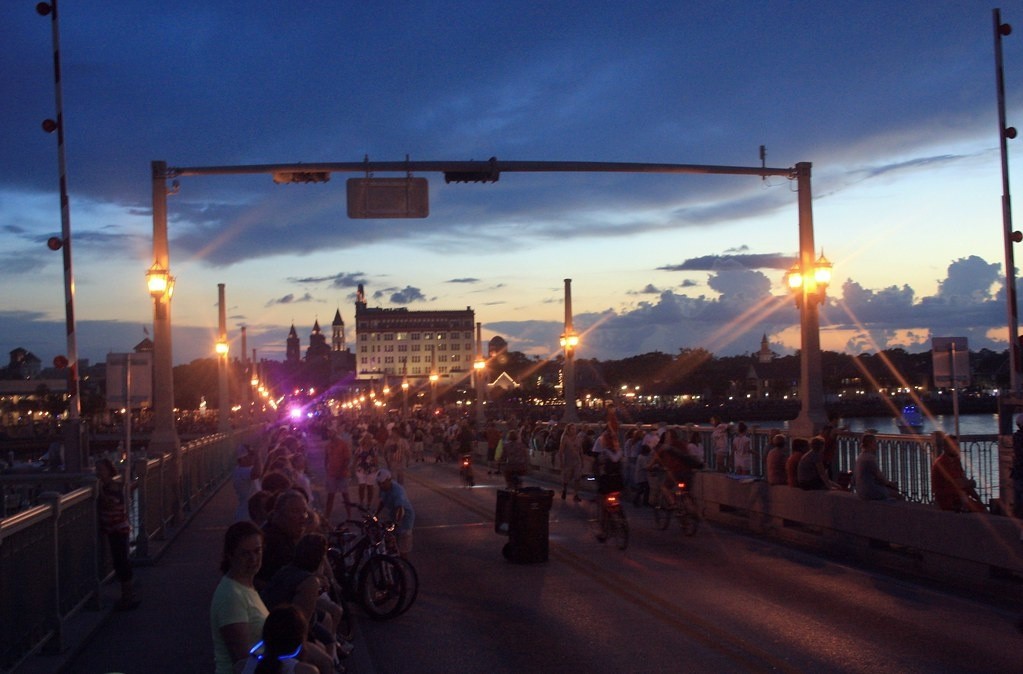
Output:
[584,474,630,550]
[320,501,417,623]
[496,460,523,492]
[644,465,701,538]
[457,441,475,490]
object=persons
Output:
[732,419,760,477]
[4,441,64,518]
[761,413,853,492]
[374,470,417,561]
[321,428,352,525]
[932,433,987,512]
[625,422,703,510]
[354,433,378,512]
[92,456,137,592]
[312,401,624,502]
[235,420,344,596]
[709,414,735,471]
[1010,414,1023,521]
[211,521,354,674]
[855,435,905,501]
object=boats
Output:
[896,402,924,435]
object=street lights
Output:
[215,284,234,434]
[558,278,582,423]
[144,161,182,460]
[402,367,410,409]
[785,161,835,429]
[427,344,440,407]
[472,321,485,408]
[383,376,391,410]
[239,327,251,429]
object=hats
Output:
[375,469,391,484]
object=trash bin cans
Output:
[494,486,555,566]
